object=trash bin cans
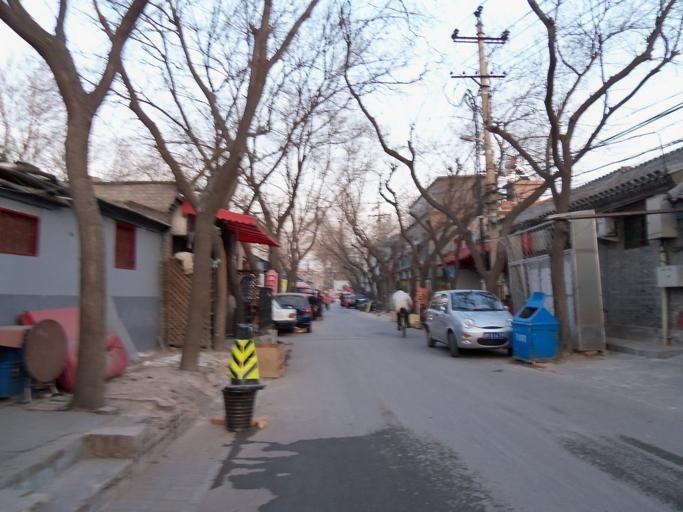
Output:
[511,292,562,359]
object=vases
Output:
[221,388,254,432]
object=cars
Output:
[339,292,367,308]
[271,293,322,333]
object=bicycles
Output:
[397,308,410,338]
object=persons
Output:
[324,293,331,310]
[392,286,414,331]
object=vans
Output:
[424,289,514,357]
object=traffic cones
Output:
[226,324,264,390]
